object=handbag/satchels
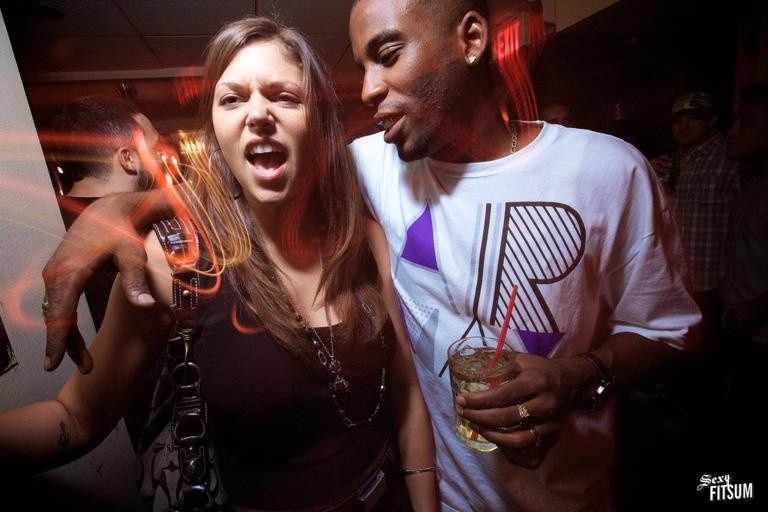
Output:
[134,218,224,512]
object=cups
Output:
[445,333,515,456]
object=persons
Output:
[54,93,167,333]
[42,0,706,511]
[0,16,440,509]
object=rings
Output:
[516,404,530,418]
[42,300,50,312]
[530,426,540,442]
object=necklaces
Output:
[508,123,520,155]
[279,285,388,429]
[268,241,352,393]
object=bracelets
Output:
[403,466,435,476]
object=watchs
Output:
[581,351,615,403]
